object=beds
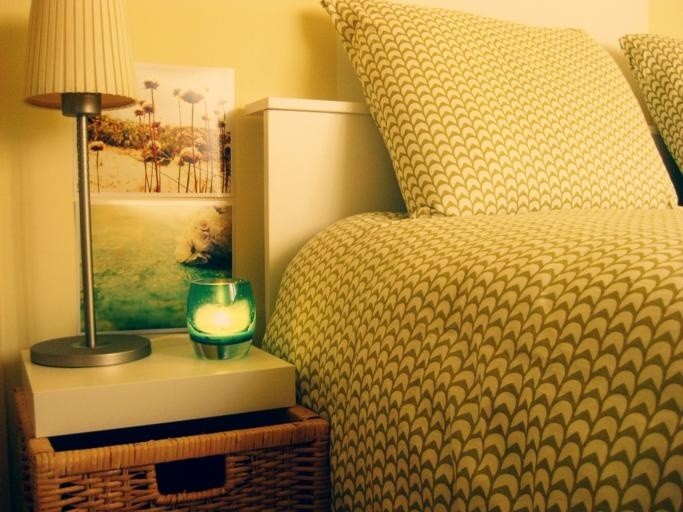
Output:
[249,95,682,512]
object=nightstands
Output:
[9,333,332,512]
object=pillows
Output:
[325,0,680,215]
[619,34,682,176]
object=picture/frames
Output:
[72,62,236,198]
[76,202,238,334]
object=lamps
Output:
[23,0,152,369]
